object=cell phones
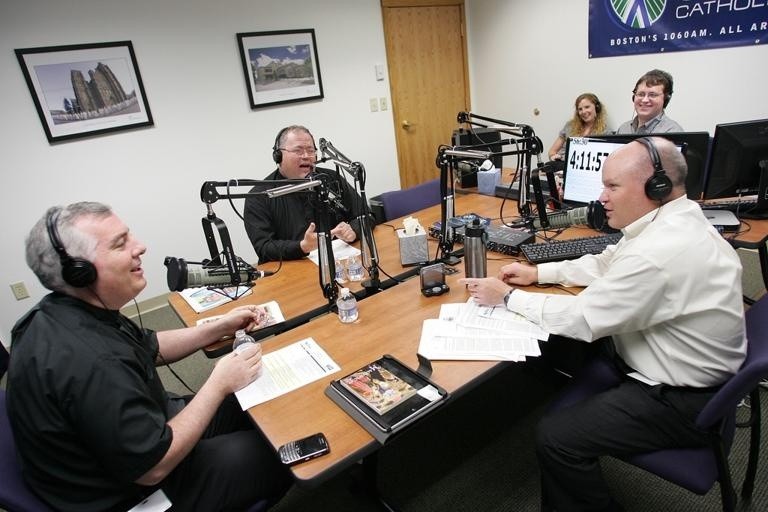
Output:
[277,432,328,466]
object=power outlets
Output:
[9,280,30,302]
[368,96,388,112]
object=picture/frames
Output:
[13,40,154,144]
[235,27,325,110]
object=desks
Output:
[214,212,630,512]
[168,192,541,359]
[446,165,768,306]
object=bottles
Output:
[336,287,359,323]
[345,255,365,282]
[549,175,564,208]
[231,330,263,379]
[333,257,347,284]
[447,215,489,288]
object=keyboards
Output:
[521,232,621,264]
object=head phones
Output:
[44,207,98,285]
[273,128,282,163]
[595,102,602,114]
[632,74,671,107]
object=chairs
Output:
[369,178,452,225]
[0,340,270,512]
[540,292,768,512]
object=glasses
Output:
[278,147,317,158]
[633,90,664,99]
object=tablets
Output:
[330,355,446,430]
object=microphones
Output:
[303,172,350,220]
[168,254,273,293]
[527,199,605,231]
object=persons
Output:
[243,126,376,265]
[468,136,748,512]
[5,201,277,511]
[615,68,684,135]
[545,94,614,172]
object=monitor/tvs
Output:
[563,119,764,231]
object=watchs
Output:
[504,288,516,306]
